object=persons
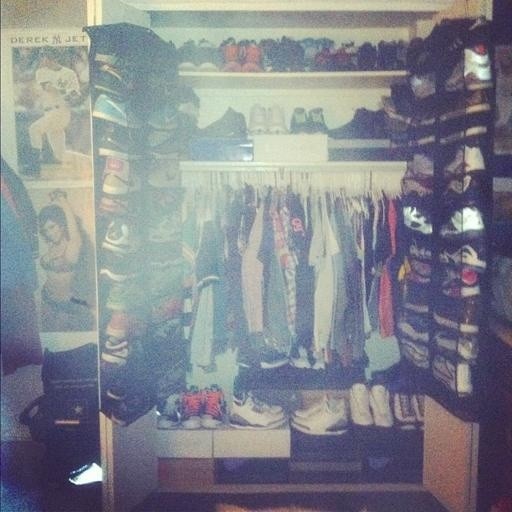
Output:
[39,187,84,320]
[18,48,92,180]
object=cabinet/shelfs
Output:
[94,2,499,424]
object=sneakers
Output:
[21,162,41,175]
[91,36,188,426]
[232,390,286,414]
[289,394,346,419]
[202,384,228,430]
[370,384,394,430]
[395,15,492,399]
[227,404,288,429]
[390,386,419,432]
[157,390,184,431]
[348,382,374,427]
[172,34,404,139]
[180,384,204,430]
[290,400,350,437]
[411,391,425,432]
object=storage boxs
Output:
[156,427,422,483]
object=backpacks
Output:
[19,343,100,452]
